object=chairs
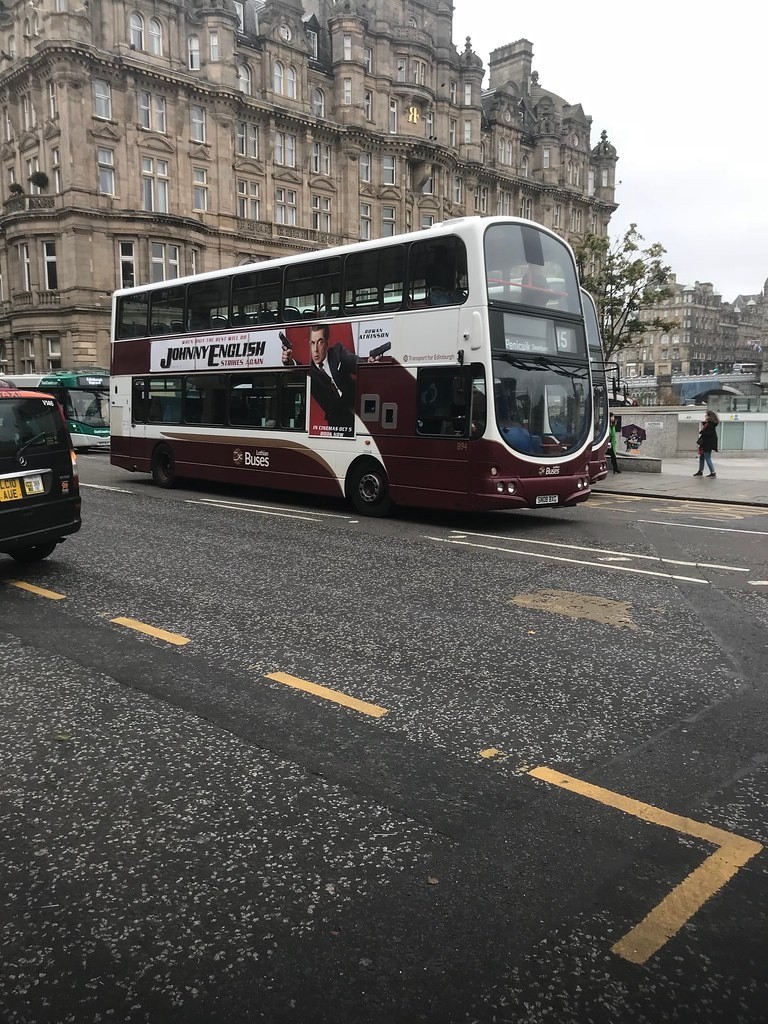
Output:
[150,284,467,335]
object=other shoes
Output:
[694,471,702,477]
[706,472,716,478]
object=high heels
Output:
[613,468,621,474]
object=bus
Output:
[732,363,757,374]
[0,370,110,453]
[110,215,628,519]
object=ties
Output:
[319,363,339,398]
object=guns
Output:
[278,331,293,351]
[369,341,391,361]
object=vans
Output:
[0,387,82,562]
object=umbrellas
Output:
[622,424,646,441]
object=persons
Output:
[624,427,642,454]
[520,262,549,305]
[693,410,718,478]
[607,413,622,474]
[282,325,381,437]
